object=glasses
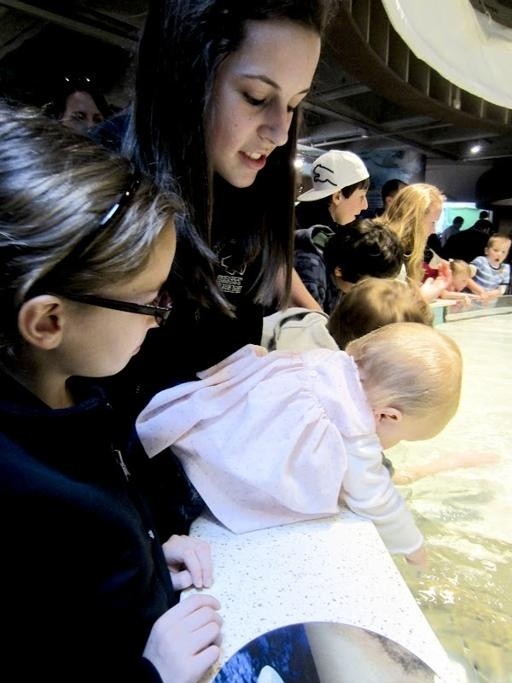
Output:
[41,282,175,329]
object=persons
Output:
[87,0,328,394]
[50,80,113,134]
[137,317,470,567]
[261,148,510,350]
[0,97,225,683]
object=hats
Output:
[296,148,370,203]
